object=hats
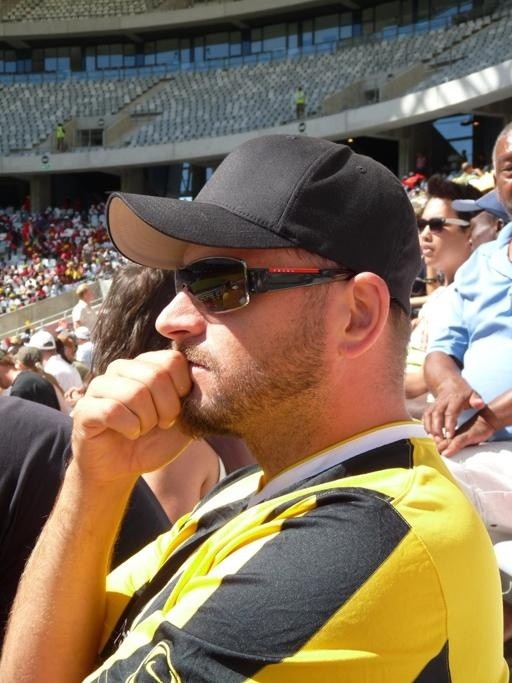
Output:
[106,135,421,315]
[75,326,90,339]
[24,331,56,350]
[453,188,510,222]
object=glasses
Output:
[417,218,468,230]
[174,255,358,314]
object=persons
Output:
[2,123,512,663]
[293,86,307,120]
